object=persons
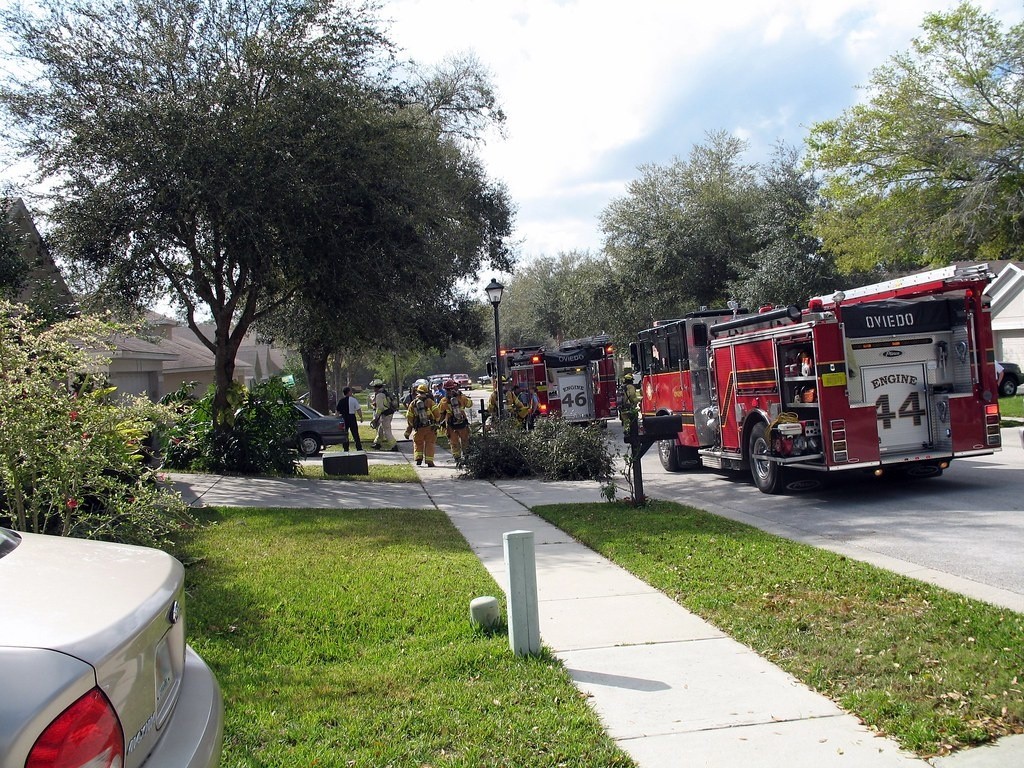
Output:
[995,360,1004,387]
[401,378,473,466]
[369,378,398,451]
[616,372,642,443]
[335,387,364,451]
[487,375,541,430]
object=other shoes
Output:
[387,445,398,451]
[428,461,434,467]
[456,458,460,461]
[370,443,380,449]
[417,459,422,465]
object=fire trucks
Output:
[630,261,1003,494]
[485,335,617,431]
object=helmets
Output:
[369,378,383,386]
[624,375,635,380]
[443,379,455,388]
[417,384,428,393]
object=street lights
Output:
[392,350,400,409]
[485,279,505,420]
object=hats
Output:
[511,385,519,391]
[496,376,506,380]
[343,387,350,395]
[436,383,441,387]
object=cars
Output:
[0,526,221,768]
[411,375,454,392]
[997,362,1024,397]
[478,373,492,384]
[452,374,472,391]
[232,402,346,457]
[297,389,336,413]
